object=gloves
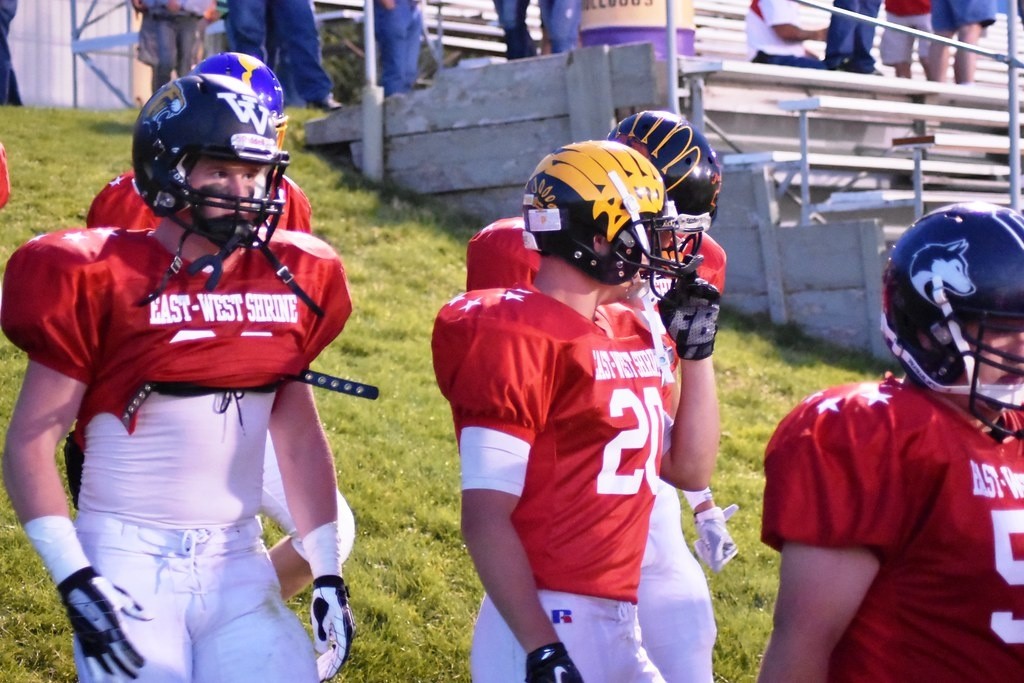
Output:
[310,575,356,681]
[526,643,584,683]
[693,504,739,572]
[658,254,721,360]
[59,566,155,679]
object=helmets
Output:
[523,141,704,286]
[188,53,289,149]
[882,203,1024,440]
[132,73,289,248]
[608,110,722,231]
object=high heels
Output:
[305,91,343,110]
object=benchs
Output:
[314,1,1024,242]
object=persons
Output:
[0,142,11,210]
[0,73,355,683]
[373,0,422,100]
[0,0,24,107]
[879,0,936,79]
[824,0,885,77]
[431,140,726,683]
[745,0,829,70]
[227,0,343,112]
[132,0,218,95]
[493,0,537,61]
[467,109,738,683]
[757,202,1024,683]
[538,0,582,54]
[929,0,997,85]
[87,52,312,239]
